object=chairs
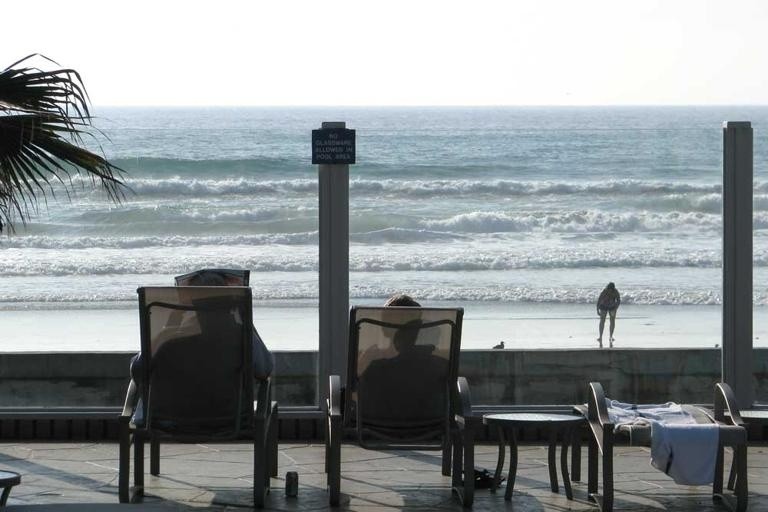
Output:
[572,382,748,511]
[325,305,476,510]
[119,285,280,511]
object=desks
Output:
[482,413,584,503]
[723,410,767,491]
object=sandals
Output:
[479,469,504,484]
[461,469,501,488]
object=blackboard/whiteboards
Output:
[312,129,356,164]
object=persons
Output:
[127,266,278,445]
[346,292,460,439]
[595,282,621,342]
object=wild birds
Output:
[492,341,506,349]
